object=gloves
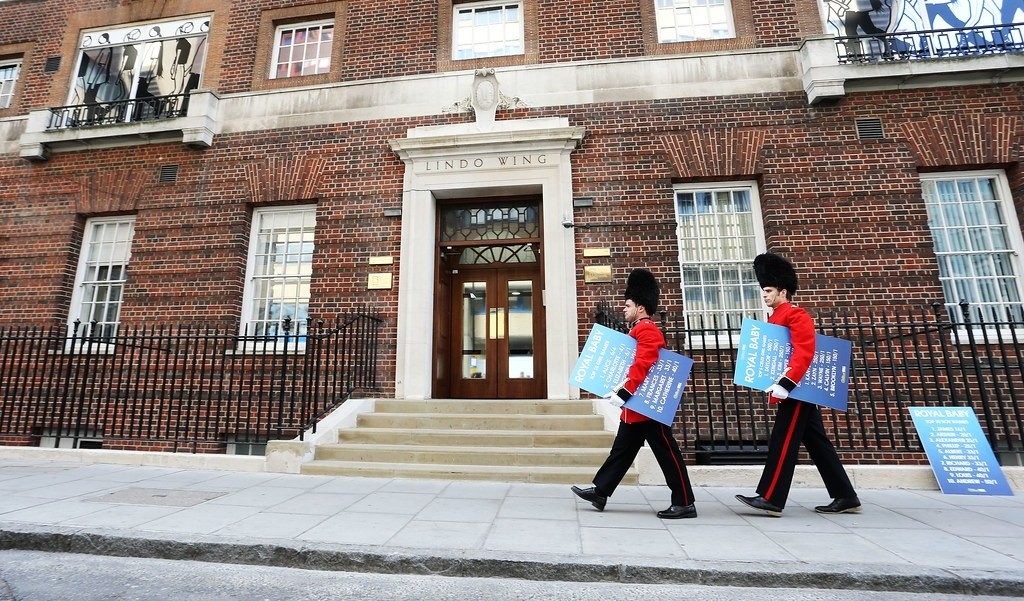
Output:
[603,391,625,407]
[764,384,789,399]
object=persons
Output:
[570,268,698,520]
[734,252,863,517]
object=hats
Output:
[753,253,798,295]
[625,268,660,314]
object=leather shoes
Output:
[571,485,607,511]
[735,495,783,517]
[815,492,862,514]
[657,503,697,519]
